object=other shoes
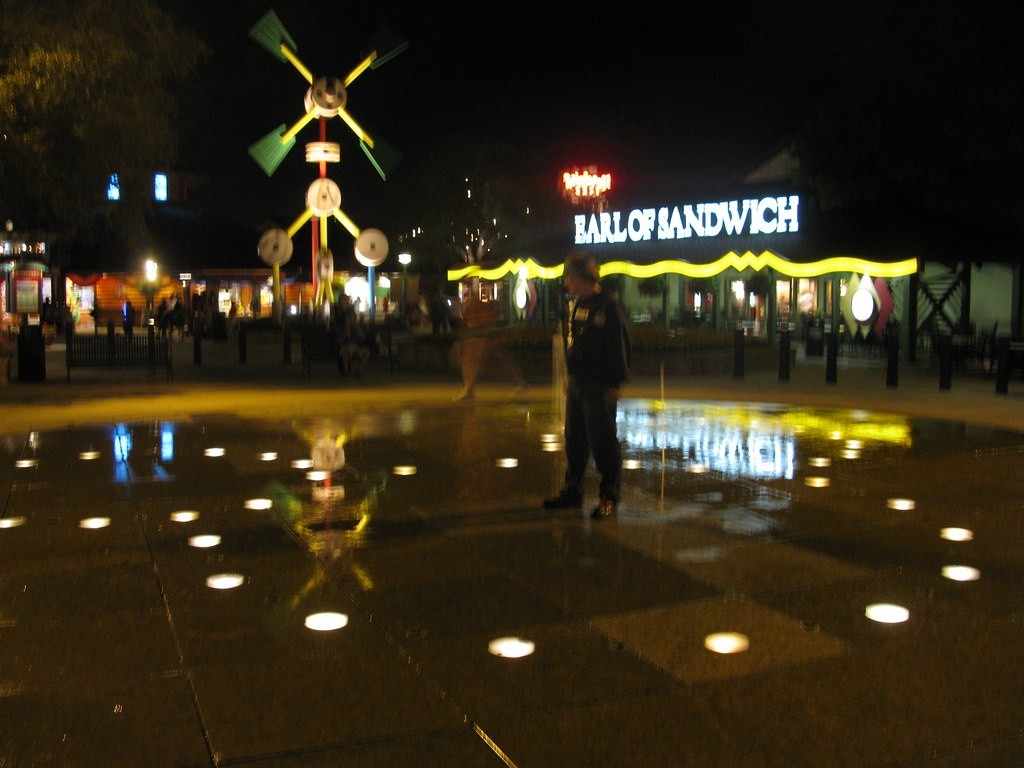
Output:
[544,496,584,508]
[592,498,621,518]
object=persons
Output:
[40,292,238,341]
[542,253,633,518]
[336,313,368,377]
[428,288,449,335]
